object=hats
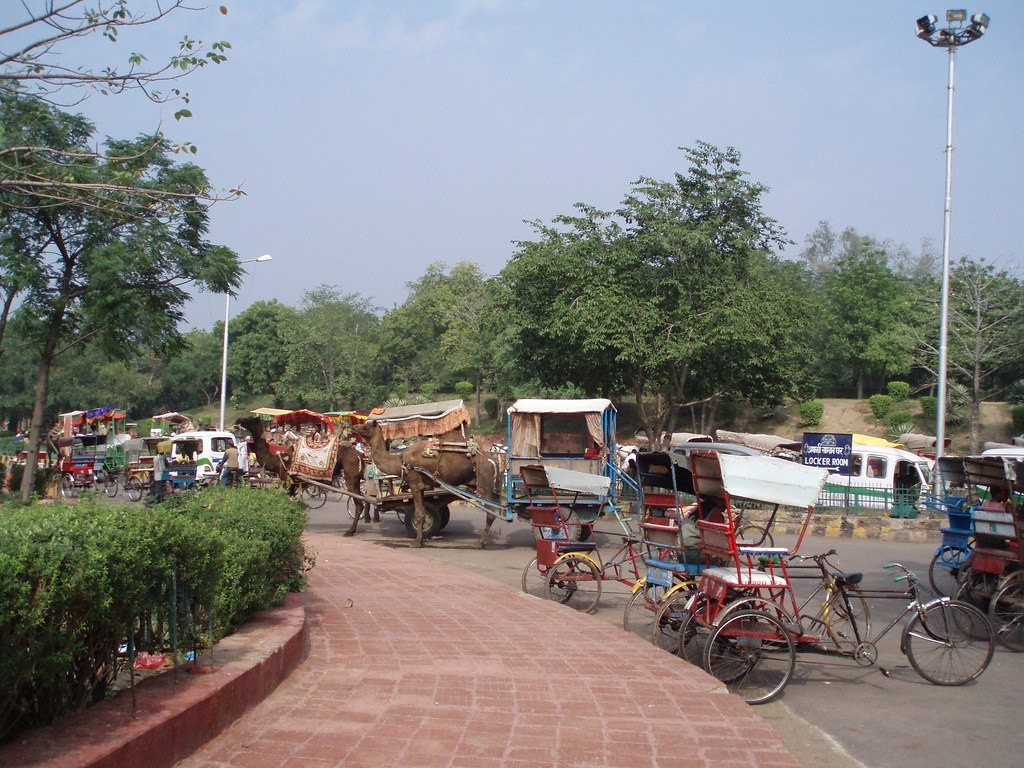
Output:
[228,439,233,444]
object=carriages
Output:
[234,399,618,549]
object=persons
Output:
[291,424,305,438]
[142,451,168,506]
[621,448,637,498]
[219,439,239,488]
[352,442,367,453]
[312,427,321,442]
[177,448,190,475]
[363,460,382,523]
[583,441,600,459]
[13,451,23,462]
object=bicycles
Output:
[346,477,407,524]
[103,466,145,501]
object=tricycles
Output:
[669,431,803,458]
[929,436,1024,651]
[44,410,265,495]
[520,451,996,704]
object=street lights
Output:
[219,254,273,431]
[916,9,991,510]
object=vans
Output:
[828,433,935,508]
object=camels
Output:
[236,414,365,537]
[348,418,507,549]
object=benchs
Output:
[129,455,156,485]
[60,456,95,472]
[696,520,804,637]
[643,514,720,608]
[971,511,1024,578]
[939,513,975,566]
[526,507,605,576]
